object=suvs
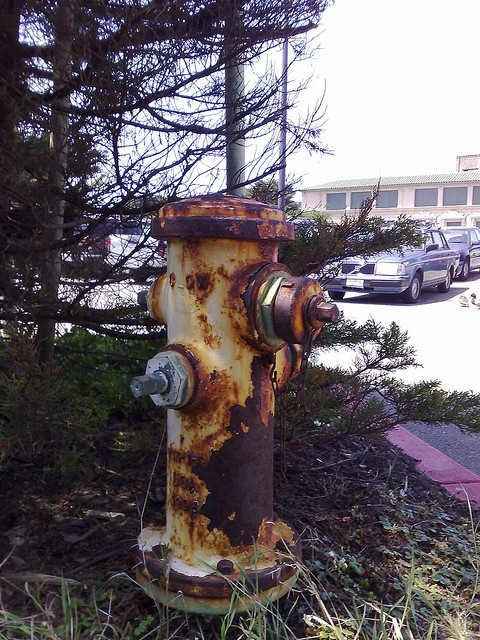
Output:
[61,214,150,284]
[139,215,167,282]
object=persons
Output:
[461,233,467,242]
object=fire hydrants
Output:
[128,193,343,619]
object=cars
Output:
[441,226,480,283]
[311,223,462,303]
